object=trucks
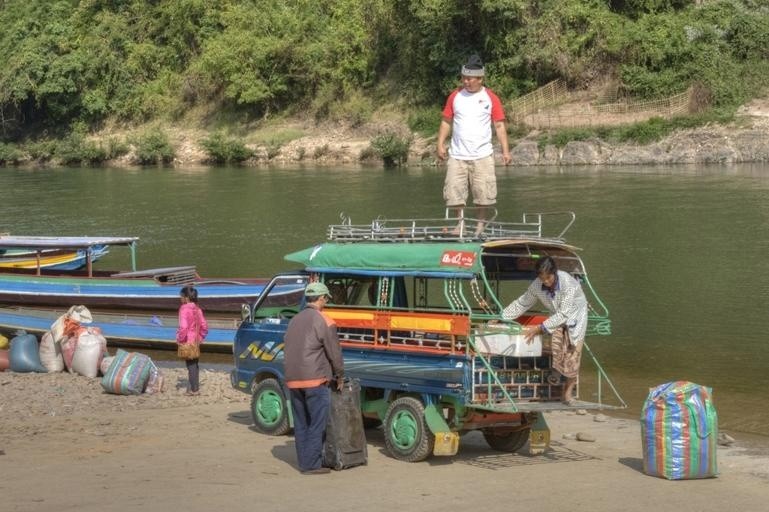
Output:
[230,204,629,463]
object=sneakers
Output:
[452,224,484,237]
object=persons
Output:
[435,57,514,237]
[283,280,346,476]
[174,286,209,397]
[485,254,590,408]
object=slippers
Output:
[562,399,578,407]
[548,374,561,387]
[185,389,200,395]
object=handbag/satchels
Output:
[641,380,718,480]
[177,342,200,359]
[100,348,157,395]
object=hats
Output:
[305,282,333,299]
[461,55,484,77]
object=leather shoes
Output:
[302,466,331,474]
